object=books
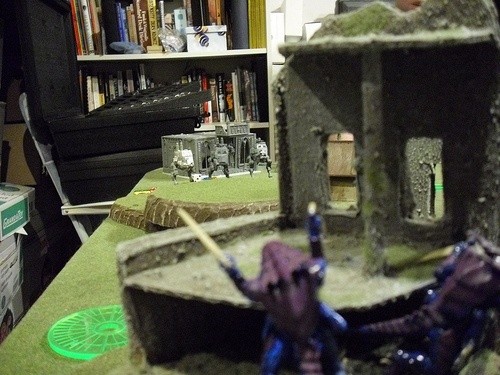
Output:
[81,61,265,124]
[70,0,267,56]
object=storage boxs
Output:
[1,226,25,314]
[0,182,36,242]
[1,273,36,344]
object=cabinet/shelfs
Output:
[67,0,271,164]
